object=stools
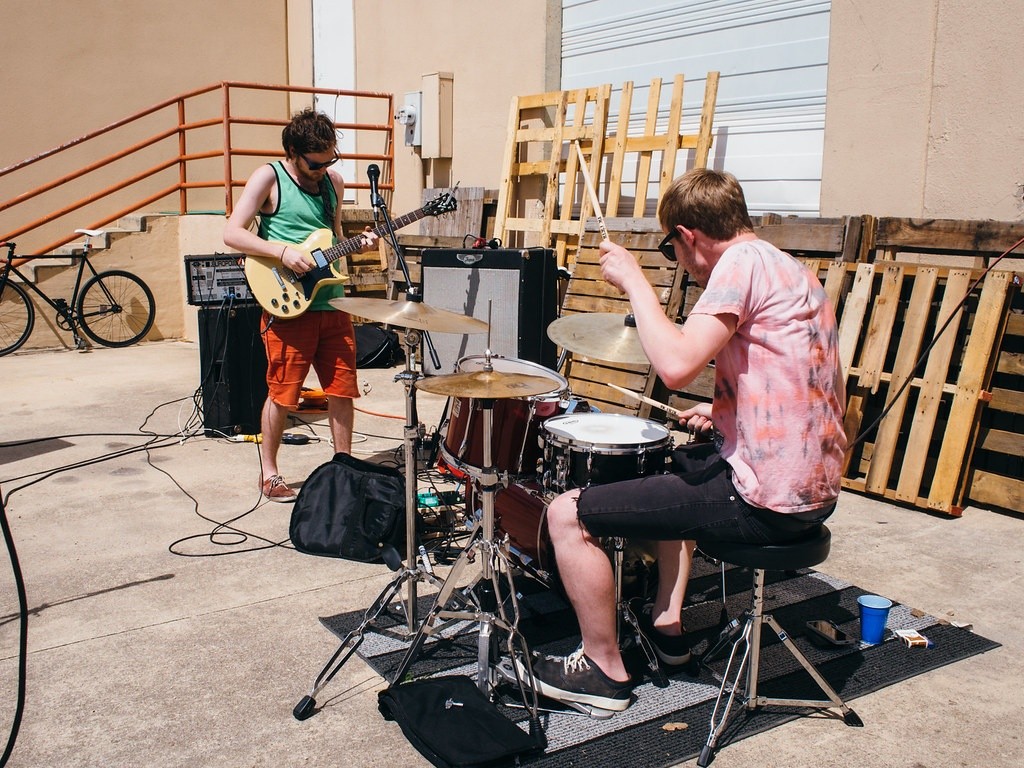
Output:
[685,524,864,768]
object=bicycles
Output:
[0,227,156,357]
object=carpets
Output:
[318,547,1002,768]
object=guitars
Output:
[243,192,458,320]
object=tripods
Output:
[291,337,547,750]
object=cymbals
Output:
[546,312,684,364]
[328,296,493,334]
[413,371,560,398]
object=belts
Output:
[755,508,833,532]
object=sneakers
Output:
[258,473,297,501]
[628,597,690,664]
[516,642,632,711]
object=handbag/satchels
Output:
[378,675,538,768]
[290,452,425,562]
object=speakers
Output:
[197,302,271,438]
[422,248,558,375]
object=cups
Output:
[857,595,893,645]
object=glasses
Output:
[296,149,339,170]
[658,223,696,260]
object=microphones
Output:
[367,164,380,221]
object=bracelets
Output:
[280,245,287,263]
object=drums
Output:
[472,476,571,601]
[442,355,571,480]
[535,412,672,498]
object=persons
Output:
[510,168,850,713]
[221,107,381,503]
